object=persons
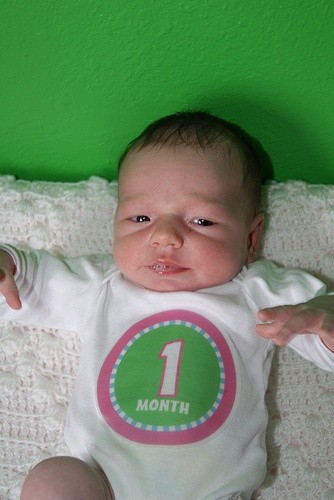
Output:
[0,111,334,500]
